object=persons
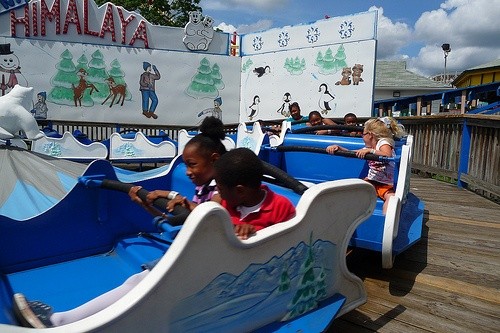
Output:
[212,147,297,241]
[284,102,310,133]
[325,116,407,215]
[341,113,363,136]
[13,115,229,330]
[379,102,392,116]
[309,110,339,137]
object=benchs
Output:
[256,128,425,270]
[0,154,377,333]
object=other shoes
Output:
[12,292,55,329]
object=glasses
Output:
[361,132,369,137]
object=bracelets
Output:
[168,191,179,200]
[371,149,376,154]
[327,129,332,134]
